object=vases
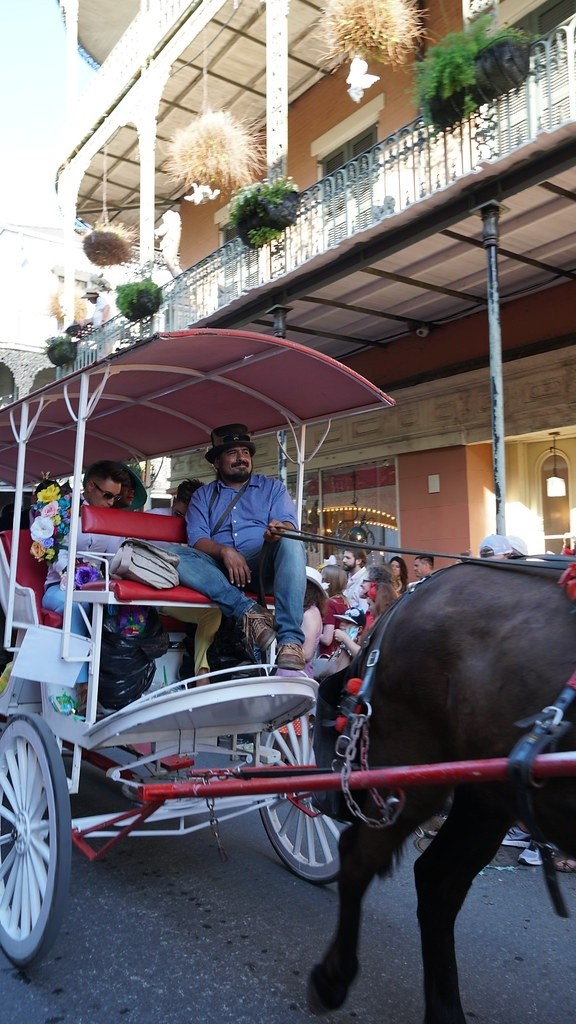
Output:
[237,191,297,249]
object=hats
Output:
[116,458,148,511]
[480,533,513,557]
[205,424,256,463]
[332,607,367,626]
[305,566,329,598]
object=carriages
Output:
[0,329,576,1024]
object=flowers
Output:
[229,175,299,248]
[29,485,72,561]
[75,562,101,590]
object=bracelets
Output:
[102,320,105,321]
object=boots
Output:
[73,684,107,715]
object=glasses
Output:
[93,482,121,502]
[174,504,183,518]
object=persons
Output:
[0,460,222,719]
[89,273,113,294]
[154,210,184,280]
[165,422,307,671]
[80,292,114,358]
[345,58,368,103]
[183,183,221,206]
[272,533,576,872]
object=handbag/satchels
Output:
[113,538,180,589]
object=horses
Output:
[303,557,575,1024]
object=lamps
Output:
[546,432,567,498]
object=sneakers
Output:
[244,603,277,653]
[278,643,305,670]
[520,842,555,866]
[501,824,532,848]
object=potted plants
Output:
[44,335,77,367]
[114,278,161,319]
[412,17,531,132]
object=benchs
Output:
[0,528,63,630]
[69,505,277,611]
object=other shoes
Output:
[554,860,576,872]
